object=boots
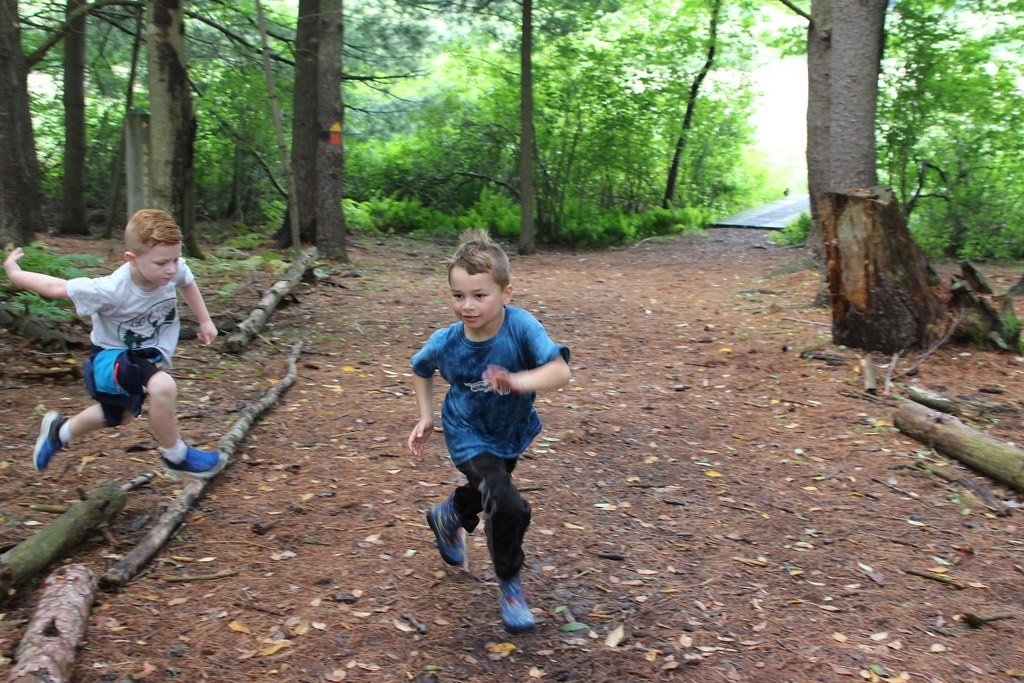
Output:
[426,487,470,566]
[496,570,535,632]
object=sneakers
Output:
[33,410,71,473]
[160,439,229,478]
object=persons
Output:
[4,209,229,477]
[408,227,570,636]
